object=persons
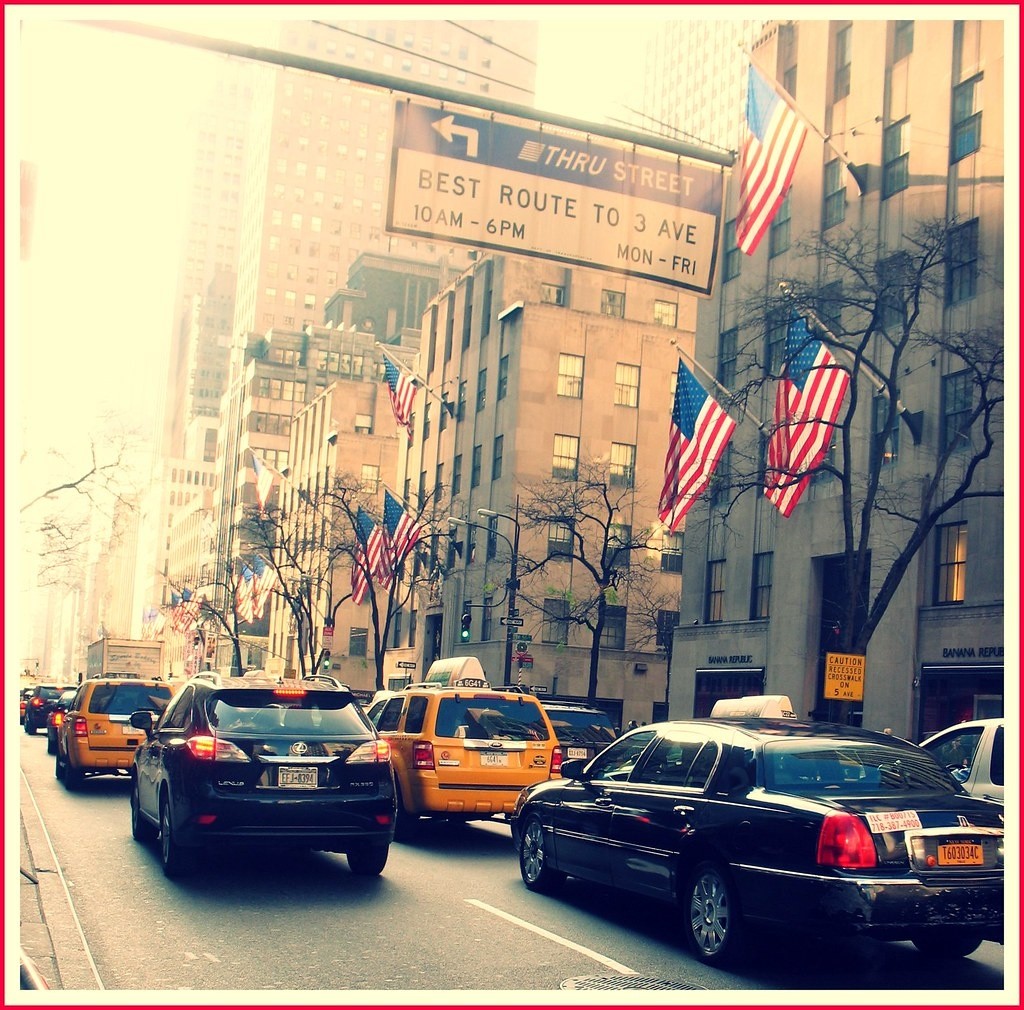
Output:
[942,736,968,764]
[276,706,313,732]
[613,720,646,737]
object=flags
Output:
[170,588,182,631]
[376,490,422,593]
[762,309,849,518]
[658,360,737,536]
[251,556,278,619]
[176,590,204,633]
[233,559,254,624]
[351,508,383,606]
[251,454,273,511]
[142,607,167,641]
[382,354,418,442]
[736,67,810,258]
